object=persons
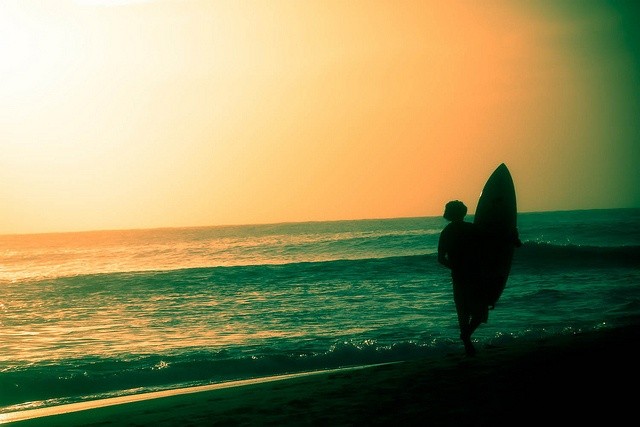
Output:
[437,199,488,358]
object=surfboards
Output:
[473,163,517,310]
